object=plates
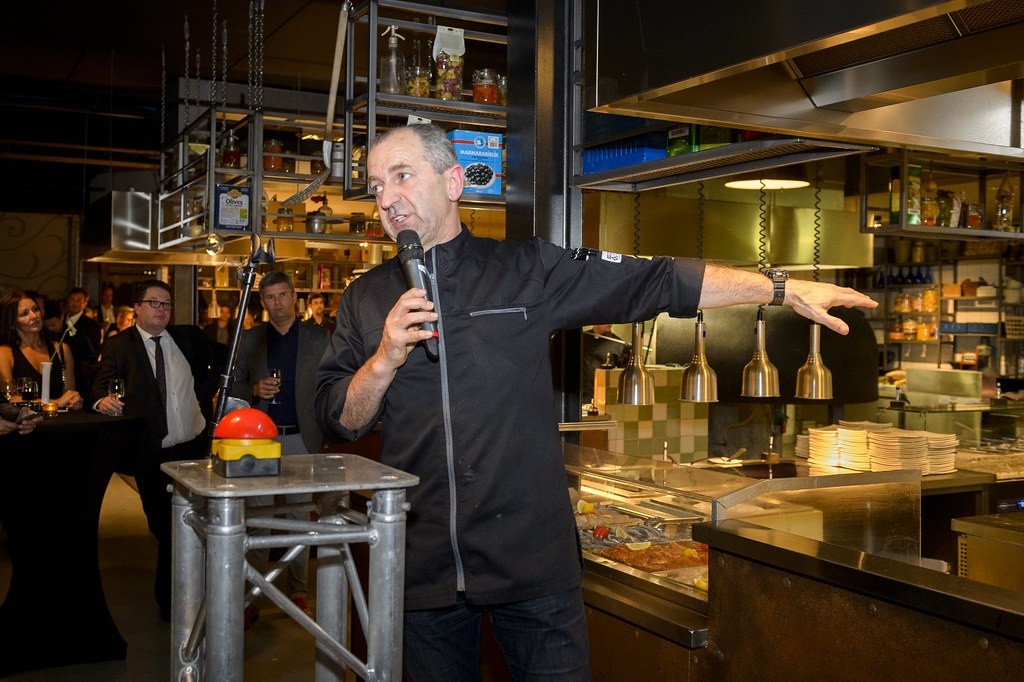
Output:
[707,457,743,464]
[795,419,960,477]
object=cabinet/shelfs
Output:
[159,0,507,250]
[834,146,1024,376]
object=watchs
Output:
[765,269,790,306]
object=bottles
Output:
[843,266,938,341]
[379,24,507,118]
[222,129,240,168]
[332,143,343,177]
[264,139,282,171]
[350,214,383,238]
[318,200,332,234]
[311,151,325,176]
[913,240,925,264]
[358,146,366,179]
[890,165,1015,232]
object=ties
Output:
[150,336,168,441]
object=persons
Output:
[204,306,237,346]
[24,285,135,374]
[228,271,330,455]
[313,123,878,682]
[0,389,43,435]
[302,293,336,333]
[92,280,220,623]
[43,303,94,366]
[0,289,84,410]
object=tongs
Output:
[643,515,704,529]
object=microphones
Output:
[397,229,438,359]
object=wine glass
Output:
[266,368,282,404]
[108,378,125,416]
[0,377,39,417]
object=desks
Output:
[0,414,144,678]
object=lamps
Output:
[614,164,832,404]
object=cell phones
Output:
[14,411,46,424]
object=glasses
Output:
[137,300,173,310]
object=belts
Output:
[276,426,301,435]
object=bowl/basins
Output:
[996,376,1024,394]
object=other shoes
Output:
[244,603,258,631]
[292,596,314,621]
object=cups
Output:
[277,208,293,232]
[42,397,59,418]
[1002,276,1024,304]
[895,240,909,264]
[306,212,326,233]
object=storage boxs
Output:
[448,130,503,197]
[213,184,252,230]
[583,112,667,174]
[906,369,981,407]
